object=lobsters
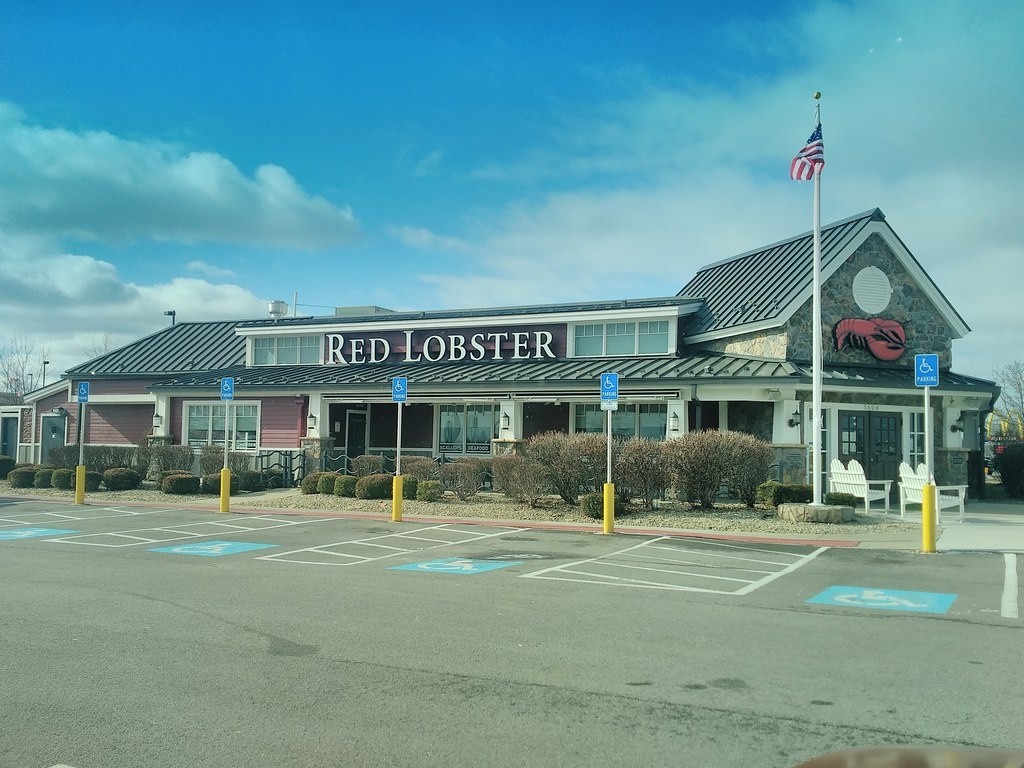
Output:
[834,317,913,362]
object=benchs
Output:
[898,462,969,523]
[828,459,894,514]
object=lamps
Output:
[153,412,162,427]
[950,416,964,433]
[670,411,679,431]
[307,412,316,429]
[788,410,801,428]
[501,412,509,429]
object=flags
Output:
[790,105,825,181]
